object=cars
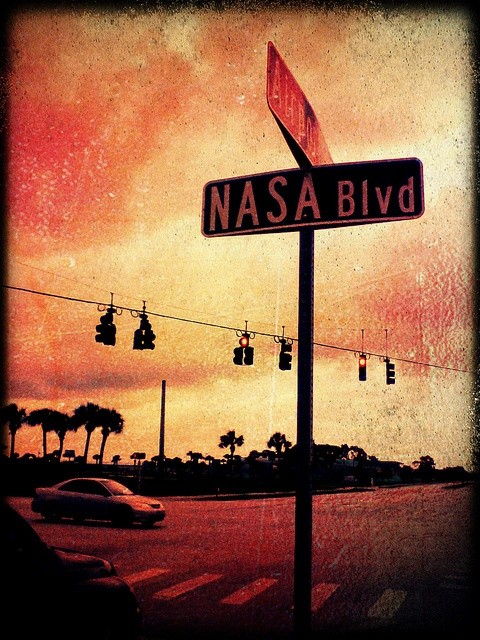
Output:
[32,476,166,527]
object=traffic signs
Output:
[266,42,334,165]
[201,158,425,238]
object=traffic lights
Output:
[134,312,156,350]
[95,305,115,345]
[274,336,294,370]
[384,357,395,384]
[233,330,255,366]
[358,355,367,381]
